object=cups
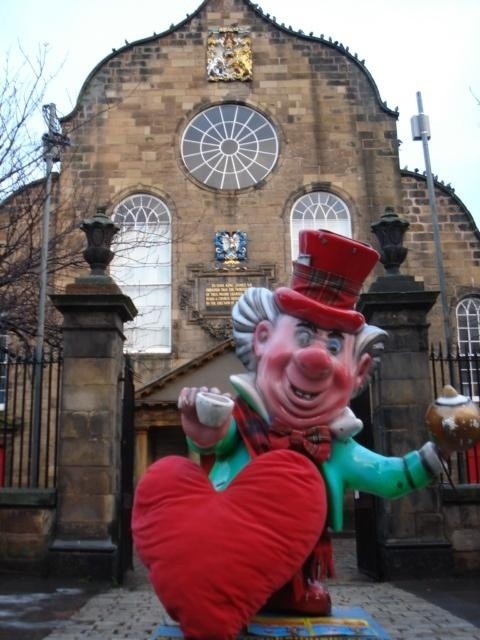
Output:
[195,391,234,429]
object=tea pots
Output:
[422,383,480,453]
